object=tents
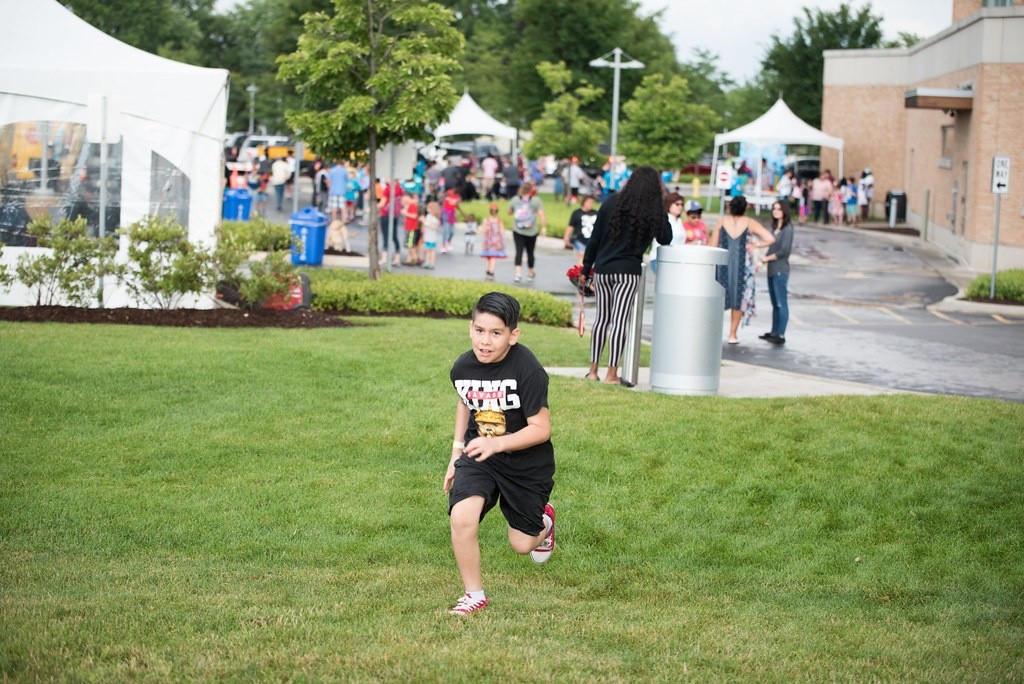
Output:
[0,0,231,309]
[705,98,843,217]
[432,84,518,170]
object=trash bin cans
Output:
[649,245,729,397]
[222,188,237,220]
[230,189,251,220]
[288,205,332,268]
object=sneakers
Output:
[759,333,785,344]
[727,338,741,343]
[530,503,556,564]
[449,593,488,616]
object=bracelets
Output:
[453,441,465,449]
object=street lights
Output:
[589,45,647,195]
[246,81,260,136]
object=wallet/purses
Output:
[578,281,585,337]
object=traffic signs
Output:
[991,156,1011,194]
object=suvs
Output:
[439,142,503,169]
[225,132,290,162]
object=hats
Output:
[685,201,704,212]
[490,204,498,209]
[864,168,871,174]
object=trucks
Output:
[255,142,370,177]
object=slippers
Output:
[585,373,599,382]
[603,376,622,384]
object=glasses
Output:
[772,208,782,211]
[673,202,683,207]
[691,216,701,219]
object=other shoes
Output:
[379,258,435,269]
[486,271,493,280]
[527,273,535,282]
[514,276,521,282]
[441,241,452,253]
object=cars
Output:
[682,161,712,175]
[434,167,525,197]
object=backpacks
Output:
[514,195,535,228]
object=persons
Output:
[564,195,598,267]
[224,147,296,216]
[709,195,776,343]
[760,200,794,344]
[579,165,673,387]
[444,291,556,615]
[730,156,874,228]
[312,154,710,282]
[33,141,60,193]
